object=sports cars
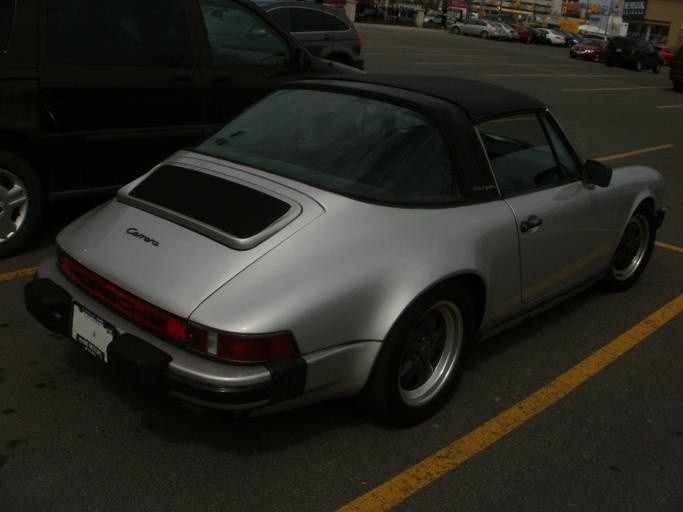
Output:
[23,75,666,432]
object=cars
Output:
[424,13,442,24]
[264,1,362,71]
[449,18,683,92]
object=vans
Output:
[0,1,366,263]
